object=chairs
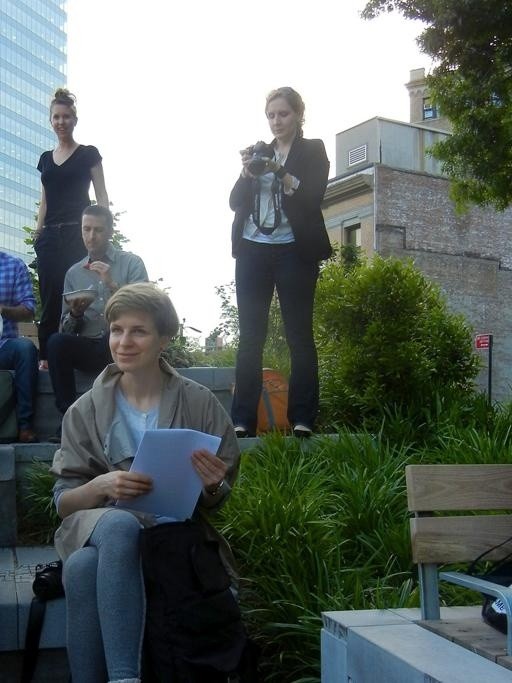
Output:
[406,461,511,674]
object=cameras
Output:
[31,558,67,599]
[247,140,275,176]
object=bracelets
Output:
[35,232,40,234]
[275,165,288,179]
[205,482,224,496]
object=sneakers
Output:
[49,431,62,443]
[19,429,40,442]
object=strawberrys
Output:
[83,263,90,269]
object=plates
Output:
[61,289,99,303]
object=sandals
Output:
[293,421,312,438]
[234,423,256,437]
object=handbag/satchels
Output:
[468,538,511,634]
[139,519,255,682]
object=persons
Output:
[0,252,38,443]
[48,282,242,683]
[229,87,332,437]
[33,88,109,371]
[47,205,148,444]
